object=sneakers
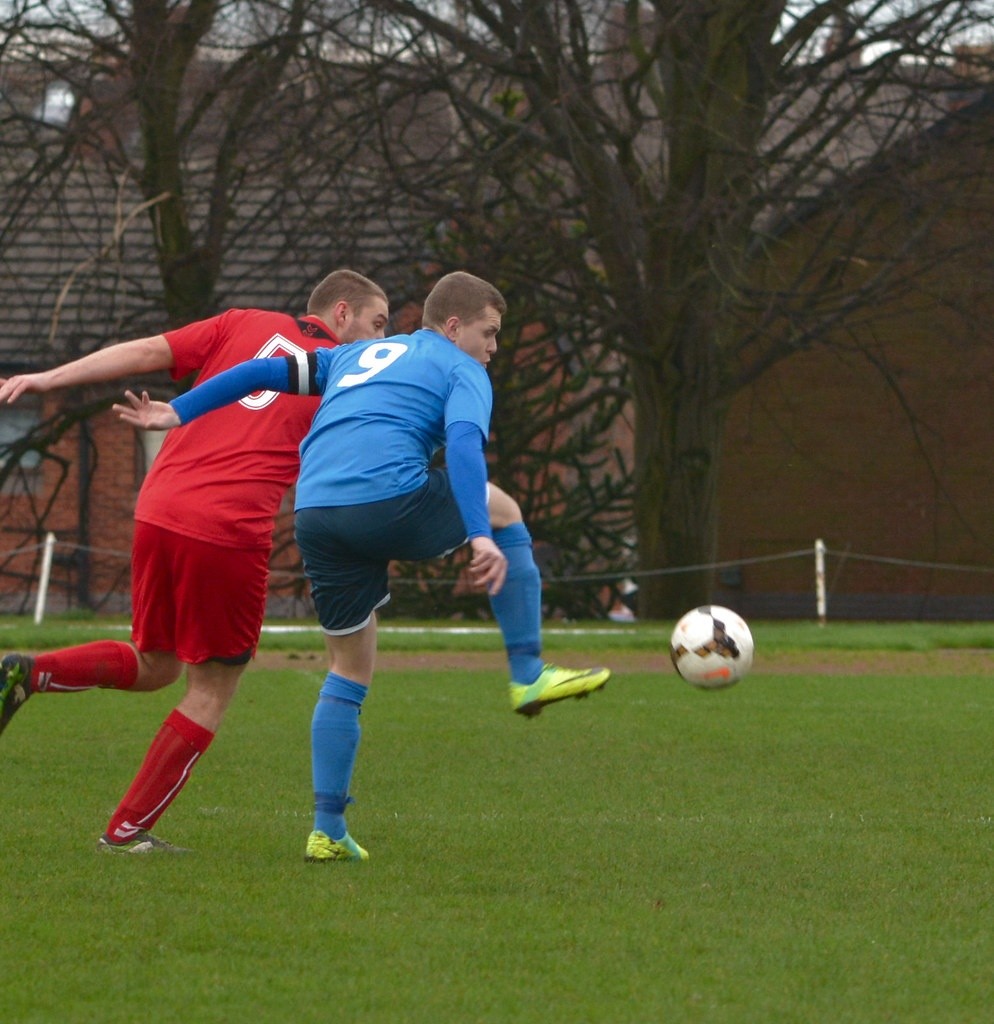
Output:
[306,829,370,863]
[508,662,611,718]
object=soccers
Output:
[669,604,754,689]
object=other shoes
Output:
[0,654,35,737]
[98,829,193,856]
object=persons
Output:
[111,272,609,862]
[0,267,389,853]
